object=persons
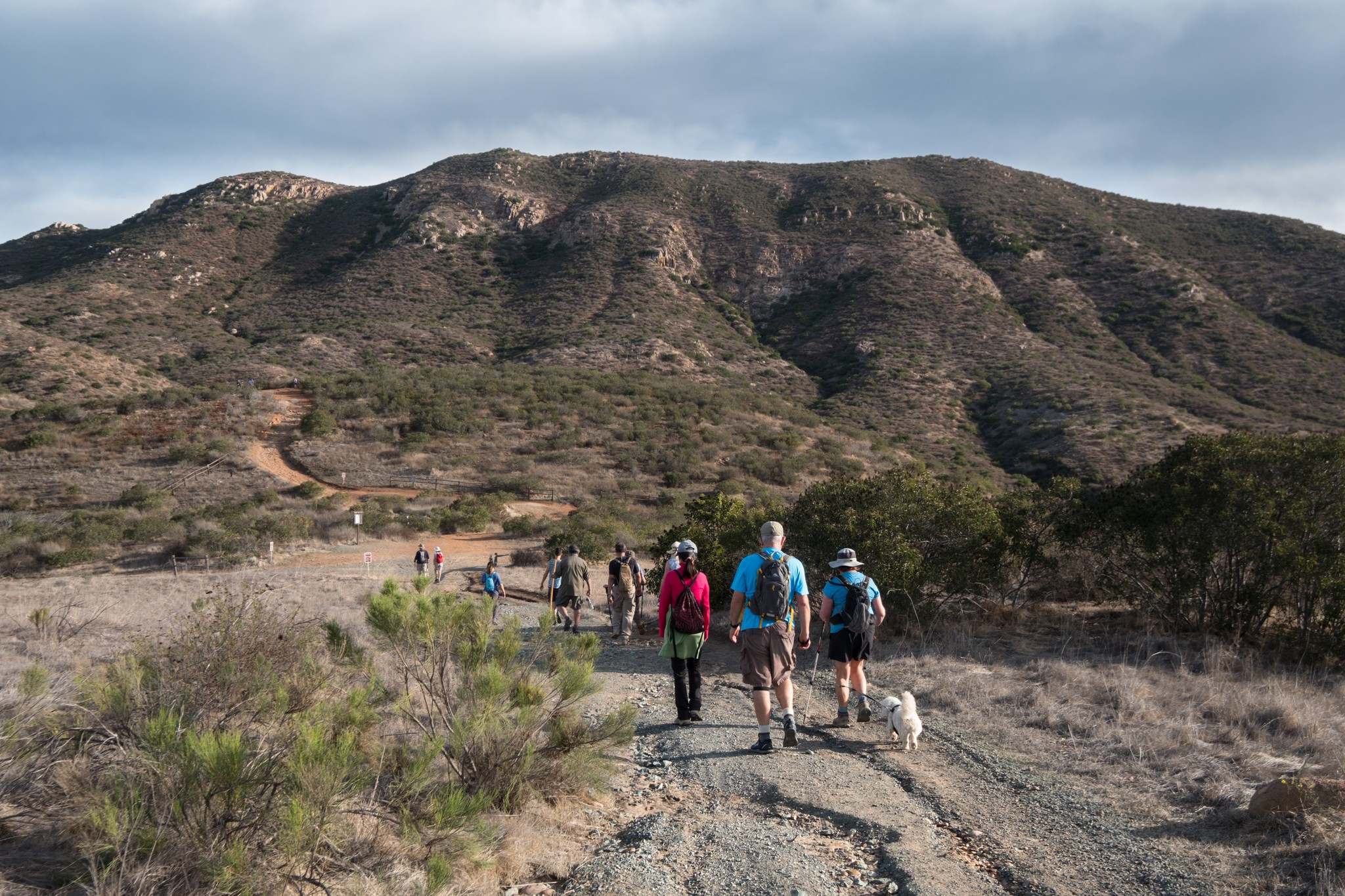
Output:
[659,540,710,725]
[540,547,562,625]
[607,542,642,645]
[603,550,646,635]
[729,521,811,753]
[414,543,429,578]
[481,562,505,625]
[433,546,443,583]
[550,545,592,633]
[820,548,885,726]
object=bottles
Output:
[586,597,594,610]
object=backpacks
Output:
[419,550,425,562]
[435,552,443,564]
[614,557,634,591]
[671,569,704,634]
[747,550,795,620]
[485,572,494,591]
[829,573,877,634]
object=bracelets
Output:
[607,593,611,595]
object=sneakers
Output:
[571,626,579,634]
[564,618,572,631]
[854,701,872,723]
[832,709,850,728]
[675,710,702,726]
[783,719,798,747]
[750,738,773,753]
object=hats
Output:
[418,544,425,549]
[677,540,697,553]
[625,550,639,561]
[761,521,783,536]
[829,548,865,568]
[434,546,440,551]
[666,541,681,554]
[488,561,495,568]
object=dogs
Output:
[880,691,924,750]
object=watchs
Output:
[730,621,739,628]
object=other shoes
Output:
[434,577,440,582]
[622,642,630,645]
[557,614,561,624]
[611,633,619,639]
[638,623,645,635]
[492,621,498,625]
[552,621,556,625]
